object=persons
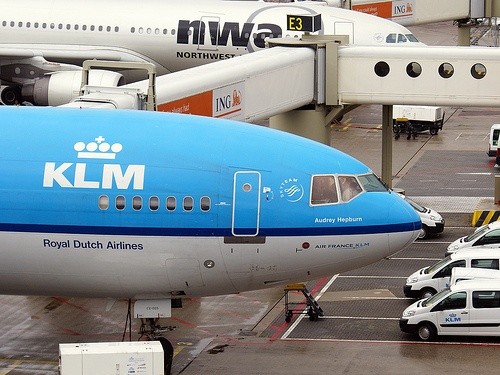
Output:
[342,181,361,202]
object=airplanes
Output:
[0,105,424,374]
[0,0,437,106]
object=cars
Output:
[399,277,500,343]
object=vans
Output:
[486,119,500,160]
[404,246,500,300]
[387,191,444,240]
[443,218,500,256]
